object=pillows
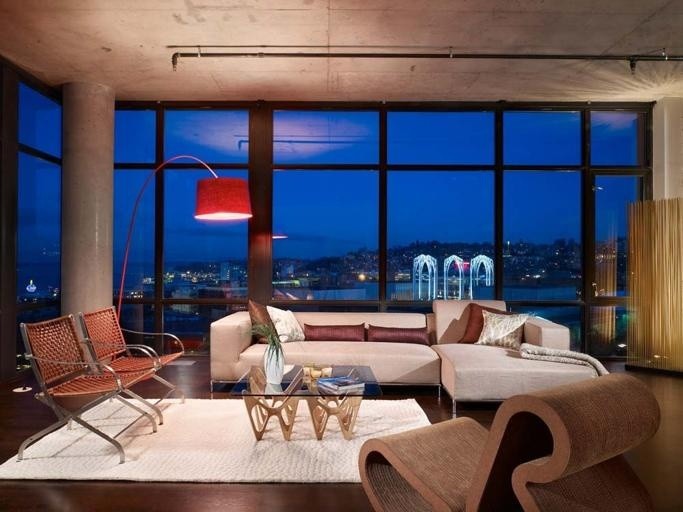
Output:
[474,310,529,351]
[368,324,430,346]
[248,299,281,345]
[457,304,517,344]
[267,305,305,343]
[304,322,365,341]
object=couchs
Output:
[210,300,598,419]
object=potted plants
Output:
[245,319,285,385]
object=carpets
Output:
[0,398,432,482]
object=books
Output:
[318,376,366,391]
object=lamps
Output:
[112,156,253,361]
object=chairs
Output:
[16,314,158,464]
[78,305,184,426]
